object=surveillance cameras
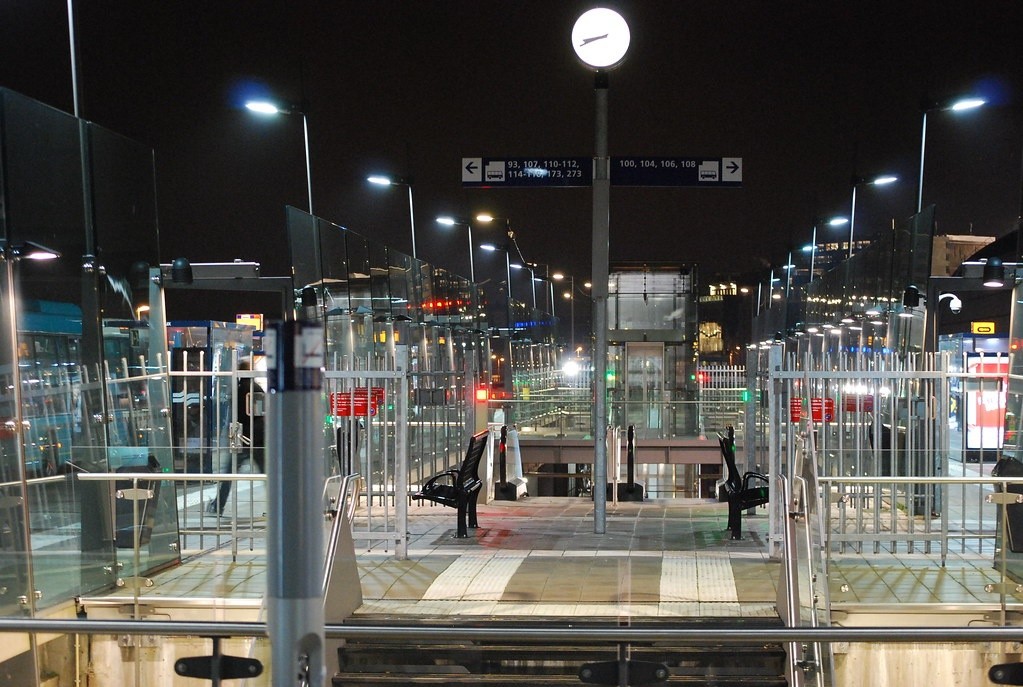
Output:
[950,299,963,314]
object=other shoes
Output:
[207,503,224,514]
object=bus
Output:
[0,297,268,506]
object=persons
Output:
[206,360,266,515]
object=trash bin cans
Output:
[990,455,1023,553]
[115,452,163,549]
[869,421,906,476]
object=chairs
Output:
[412,428,490,538]
[715,431,770,541]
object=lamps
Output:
[171,258,193,285]
[983,257,1005,289]
[300,286,318,307]
[12,240,60,261]
[394,313,412,323]
[939,293,962,313]
[775,331,794,344]
[372,316,390,323]
[795,322,808,335]
[902,284,927,310]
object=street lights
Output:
[903,93,987,286]
[767,264,796,342]
[246,101,313,216]
[551,273,575,355]
[782,244,818,340]
[436,217,475,283]
[841,176,899,319]
[584,281,593,362]
[480,245,511,298]
[756,278,781,344]
[803,217,849,333]
[366,176,416,258]
